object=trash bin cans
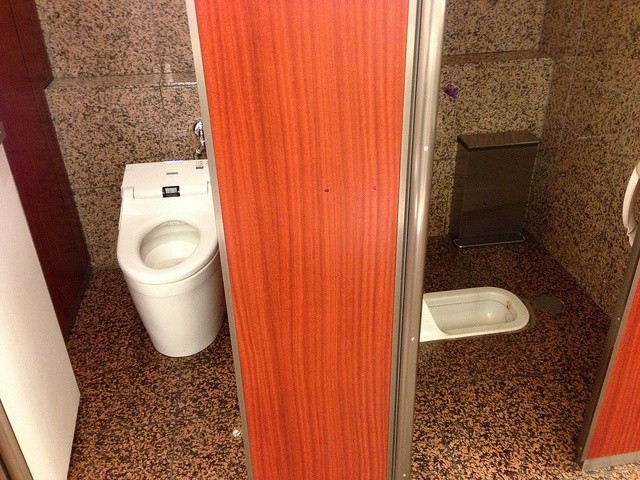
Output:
[451,128,540,248]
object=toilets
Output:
[115,159,226,358]
